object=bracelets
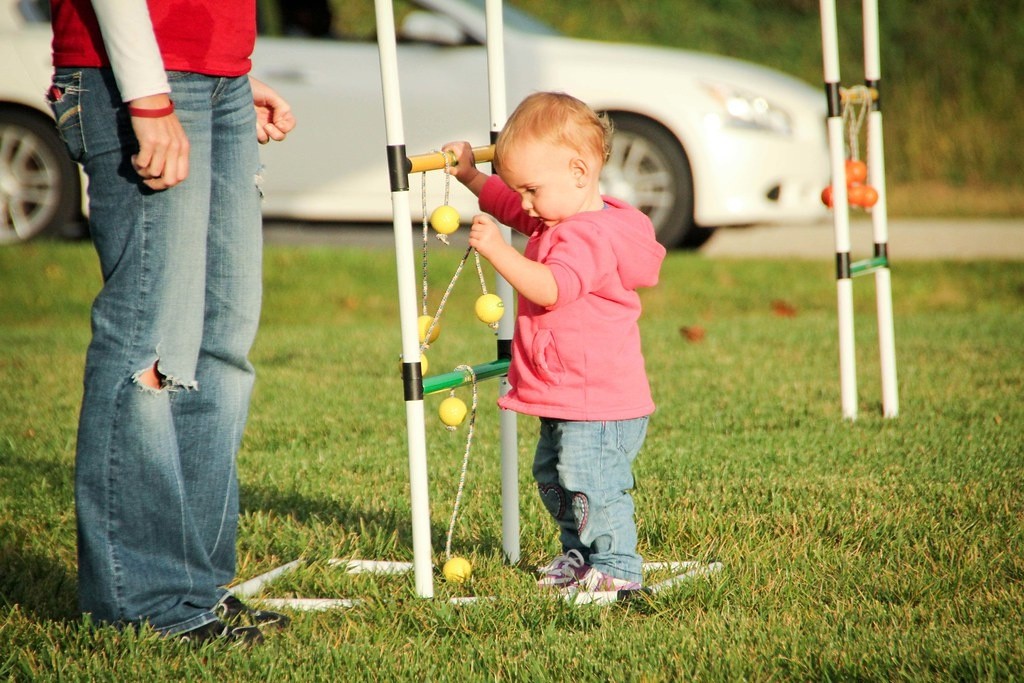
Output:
[129,99,174,118]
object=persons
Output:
[46,0,298,648]
[441,93,666,594]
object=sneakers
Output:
[169,596,291,656]
[535,548,642,606]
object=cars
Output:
[0,0,833,253]
